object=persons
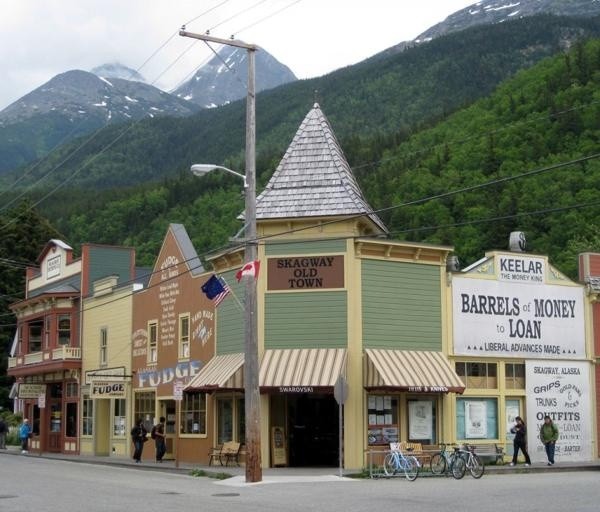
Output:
[540,416,558,465]
[509,416,531,466]
[19,418,33,454]
[0,416,9,449]
[131,418,147,462]
[154,416,167,463]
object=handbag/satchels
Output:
[151,423,163,440]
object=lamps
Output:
[193,420,199,433]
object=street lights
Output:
[191,163,262,482]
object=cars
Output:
[76,416,200,437]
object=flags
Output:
[234,261,260,282]
[201,275,224,300]
[212,277,229,306]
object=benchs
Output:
[206,439,243,466]
[463,442,506,465]
[389,440,432,466]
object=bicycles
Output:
[429,443,467,480]
[450,443,485,480]
[383,442,421,481]
